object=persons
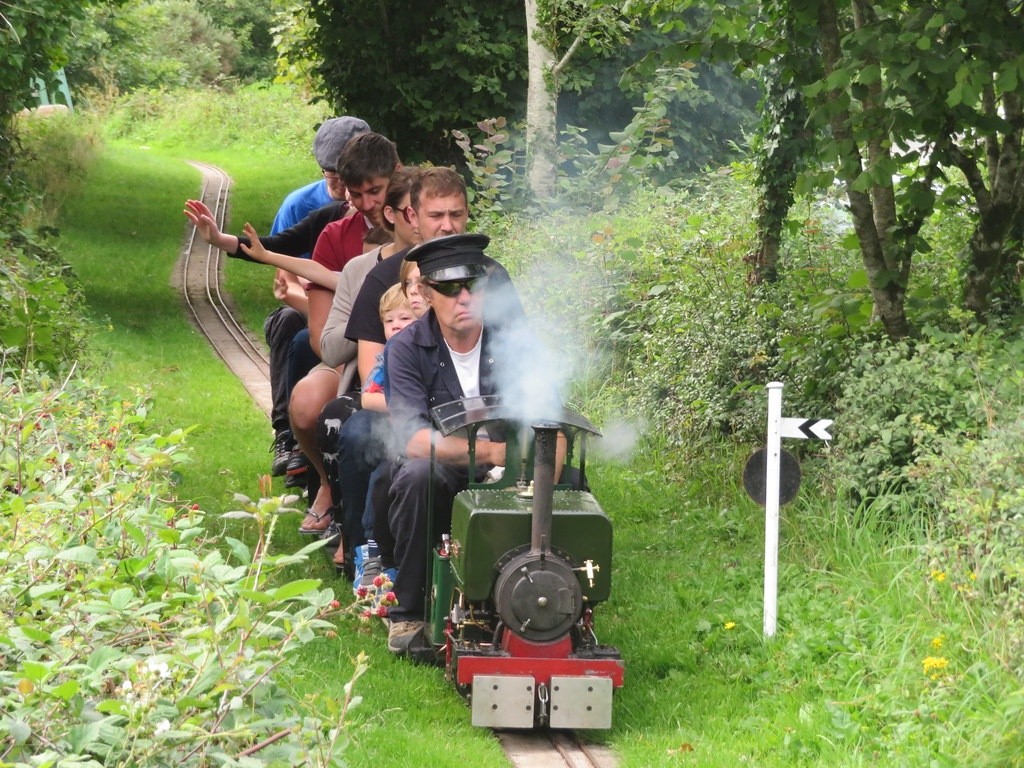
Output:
[177,118,524,618]
[385,231,587,657]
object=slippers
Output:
[298,505,335,534]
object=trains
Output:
[408,395,624,729]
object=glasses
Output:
[320,168,344,180]
[421,279,484,297]
[395,204,411,224]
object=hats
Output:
[404,232,490,280]
[313,115,371,172]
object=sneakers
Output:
[285,443,310,475]
[361,554,383,590]
[373,569,397,615]
[353,543,372,591]
[388,607,427,652]
[270,430,294,478]
[321,519,343,547]
